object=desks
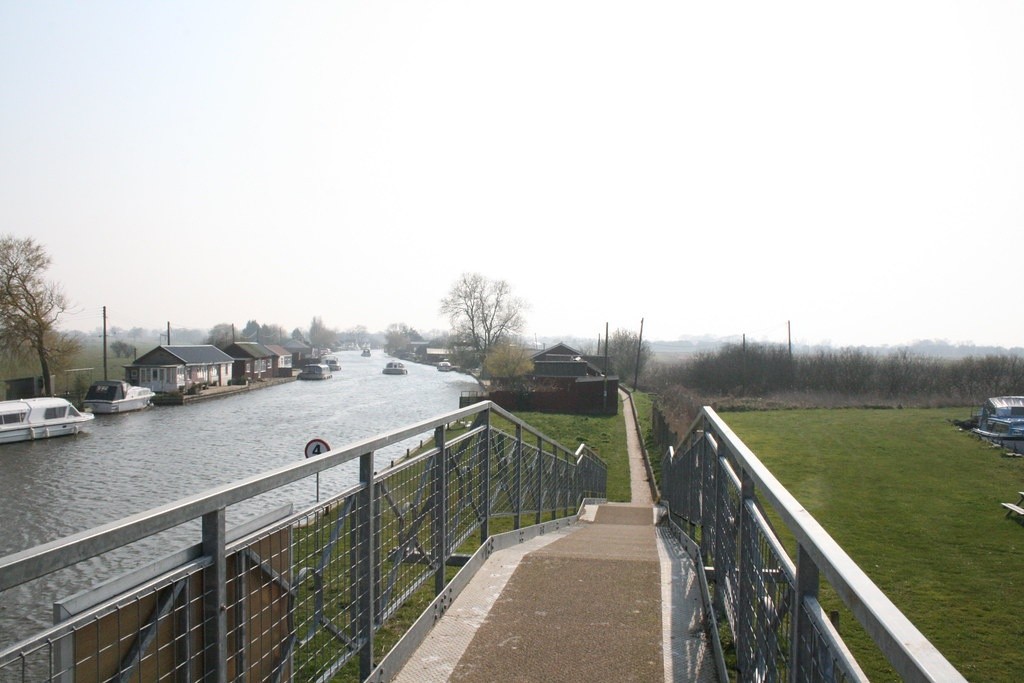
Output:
[1006,492,1024,517]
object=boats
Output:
[360,349,370,357]
[82,380,157,415]
[322,355,342,371]
[437,360,453,371]
[297,363,332,379]
[382,360,408,376]
[0,395,96,446]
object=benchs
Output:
[1001,502,1024,516]
